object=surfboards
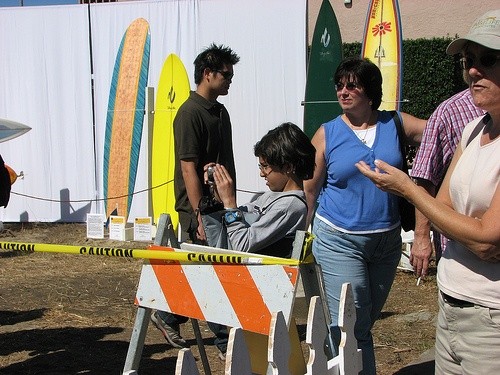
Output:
[100,17,151,218]
[304,0,346,143]
[364,0,404,112]
[150,51,190,232]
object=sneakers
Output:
[213,334,230,362]
[151,312,191,349]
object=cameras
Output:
[207,166,215,185]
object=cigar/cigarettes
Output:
[411,271,425,291]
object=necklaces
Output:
[351,114,375,144]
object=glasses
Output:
[211,67,237,80]
[458,50,500,70]
[258,162,278,174]
[334,80,361,92]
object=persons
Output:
[409,56,487,290]
[355,9,500,375]
[201,122,316,258]
[301,57,429,374]
[152,44,238,361]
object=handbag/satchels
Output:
[390,111,417,232]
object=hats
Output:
[445,9,500,56]
